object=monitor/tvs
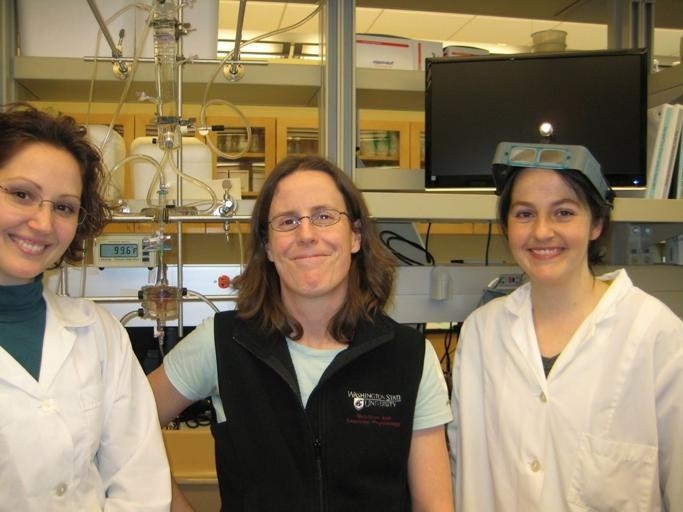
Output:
[424,46,649,195]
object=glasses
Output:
[265,208,350,231]
[0,184,88,225]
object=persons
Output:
[147,153,456,511]
[0,103,174,511]
[445,143,683,512]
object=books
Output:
[615,101,682,199]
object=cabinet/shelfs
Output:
[59,112,206,199]
[205,116,319,196]
[358,121,425,169]
